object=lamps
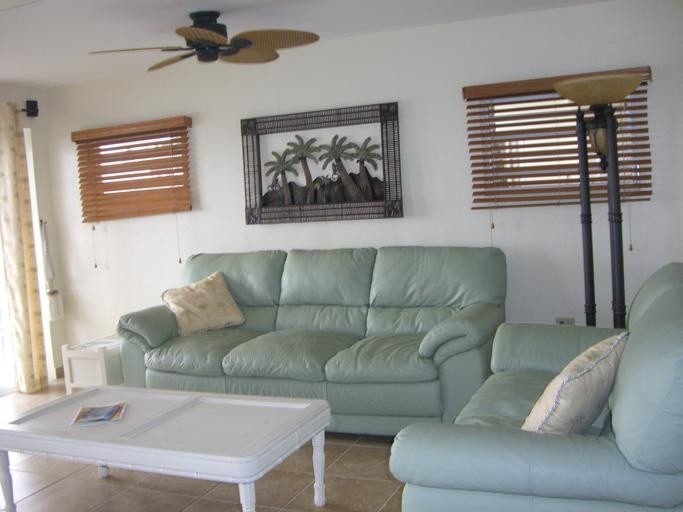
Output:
[553,70,643,326]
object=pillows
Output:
[518,332,629,439]
[160,272,245,338]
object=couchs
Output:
[388,260,681,512]
[113,246,504,437]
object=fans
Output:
[87,9,320,76]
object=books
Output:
[70,399,126,424]
[79,404,121,422]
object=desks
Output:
[61,338,121,395]
